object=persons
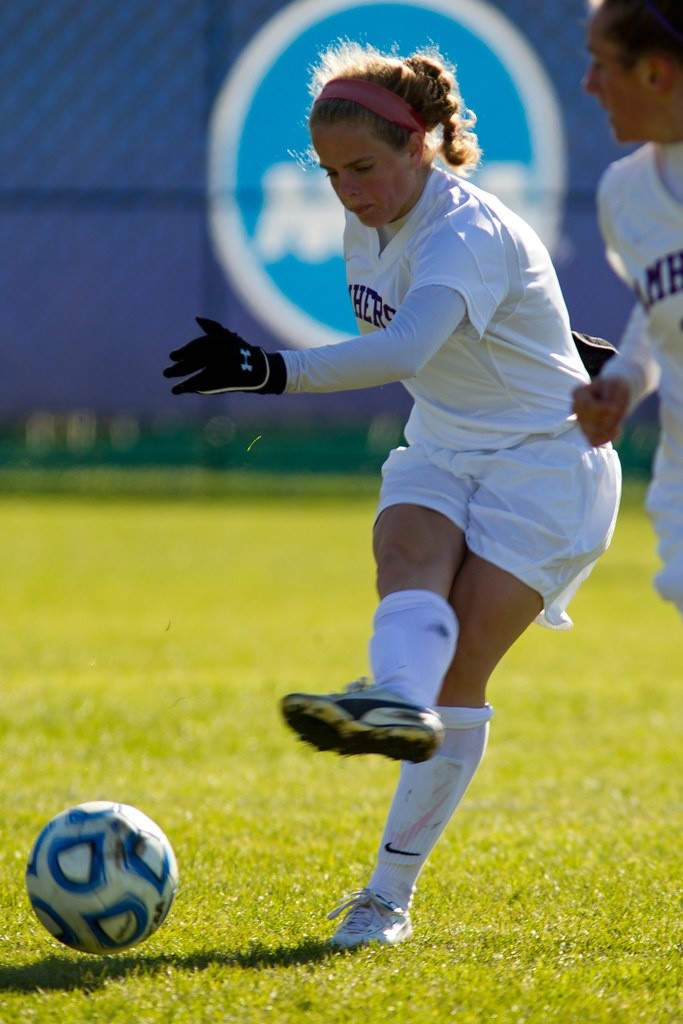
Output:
[162,53,622,951]
[571,0,682,614]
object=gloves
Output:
[162,317,288,398]
[571,331,616,376]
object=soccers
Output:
[24,800,181,956]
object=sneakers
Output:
[325,888,414,951]
[280,678,442,762]
